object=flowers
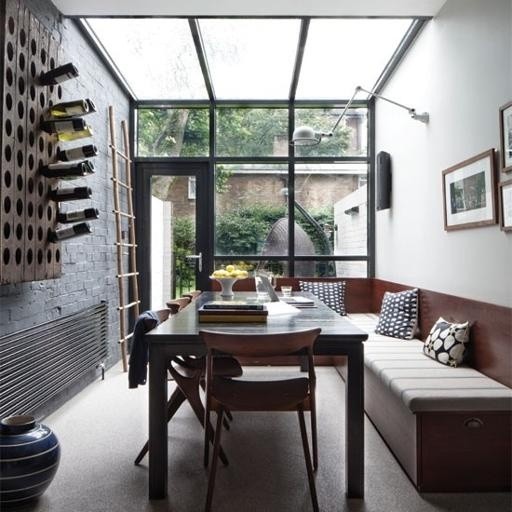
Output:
[209,258,248,279]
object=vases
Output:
[214,277,236,296]
[0,416,61,509]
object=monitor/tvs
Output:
[260,274,314,305]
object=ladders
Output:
[109,106,142,372]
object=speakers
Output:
[376,152,391,211]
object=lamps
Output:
[286,85,428,146]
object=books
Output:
[197,300,268,323]
[279,296,319,309]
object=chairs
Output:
[195,329,325,512]
[133,290,243,471]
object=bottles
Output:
[37,62,100,242]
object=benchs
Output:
[333,278,510,494]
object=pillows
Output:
[297,279,347,318]
[424,317,472,367]
[375,288,419,341]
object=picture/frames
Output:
[442,101,512,233]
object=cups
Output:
[281,285,292,294]
[254,269,277,296]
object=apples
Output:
[211,264,248,277]
